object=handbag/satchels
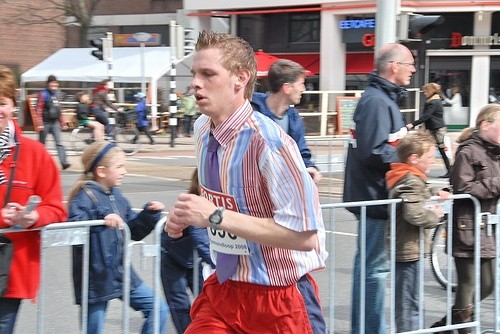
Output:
[0,145,20,295]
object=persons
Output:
[429,102,500,334]
[68,139,171,334]
[440,82,463,108]
[125,91,155,144]
[406,82,452,177]
[385,126,450,334]
[75,92,105,142]
[0,63,67,333]
[342,41,416,333]
[88,78,124,135]
[35,74,74,169]
[179,85,199,137]
[164,30,327,333]
[162,167,217,332]
[250,59,321,185]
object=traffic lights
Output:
[89,37,112,62]
[400,9,446,43]
[175,25,194,58]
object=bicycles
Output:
[71,109,141,156]
[429,187,458,292]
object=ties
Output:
[204,136,238,285]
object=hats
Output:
[133,92,146,99]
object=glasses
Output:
[387,61,416,67]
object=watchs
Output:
[210,206,225,229]
